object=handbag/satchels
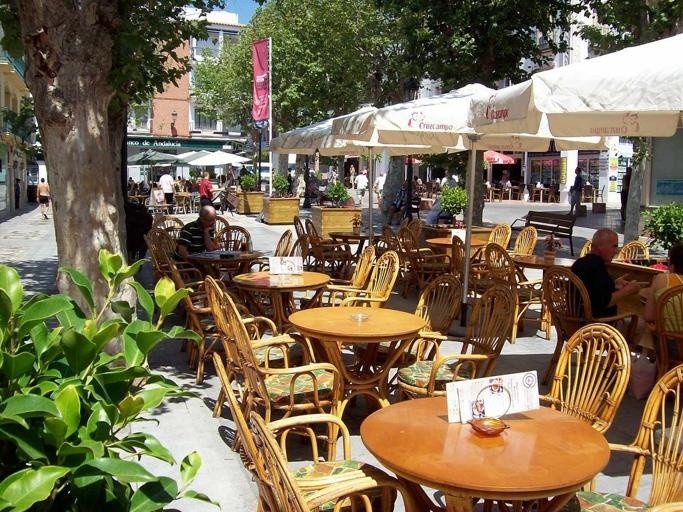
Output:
[628,351,657,400]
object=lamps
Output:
[170,110,177,129]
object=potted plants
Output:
[641,203,682,275]
[237,176,263,214]
[424,187,491,262]
[262,175,300,223]
[308,179,362,238]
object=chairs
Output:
[127,188,201,215]
[210,351,398,511]
[395,208,491,346]
[554,363,682,511]
[222,293,342,480]
[414,184,605,203]
[491,219,682,347]
[534,322,631,492]
[397,285,517,399]
[249,407,414,511]
[205,275,313,419]
[144,210,402,386]
[361,275,463,395]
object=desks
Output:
[288,306,426,451]
[359,395,612,512]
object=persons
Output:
[127,172,214,272]
[37,178,50,221]
[14,178,21,210]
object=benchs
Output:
[507,208,578,256]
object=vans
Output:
[237,161,295,184]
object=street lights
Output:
[257,128,262,191]
